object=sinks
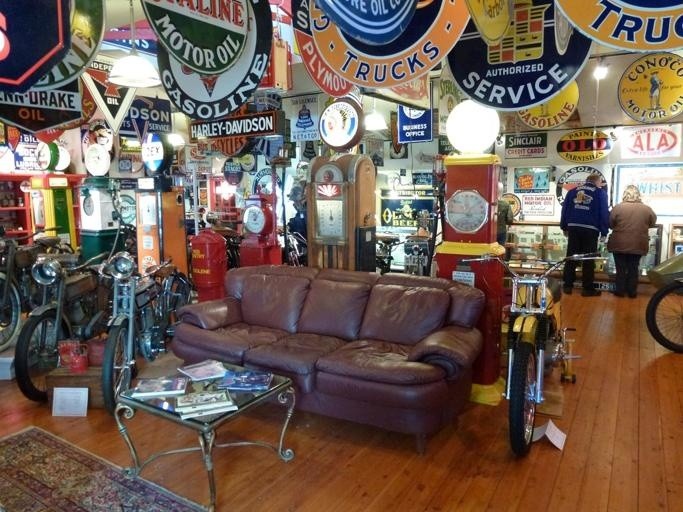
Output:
[170,263,487,456]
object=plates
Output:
[506,221,663,283]
[0,172,32,244]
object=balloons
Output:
[443,187,490,236]
[314,199,348,241]
[240,205,272,234]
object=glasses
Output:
[106,1,161,89]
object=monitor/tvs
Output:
[130,357,276,420]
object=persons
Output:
[497,181,514,245]
[607,184,658,298]
[559,172,610,297]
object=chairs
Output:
[562,281,603,297]
[609,287,637,297]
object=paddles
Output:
[0,424,206,512]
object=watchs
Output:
[0,225,194,415]
[374,210,436,273]
[644,253,683,353]
[463,251,608,455]
